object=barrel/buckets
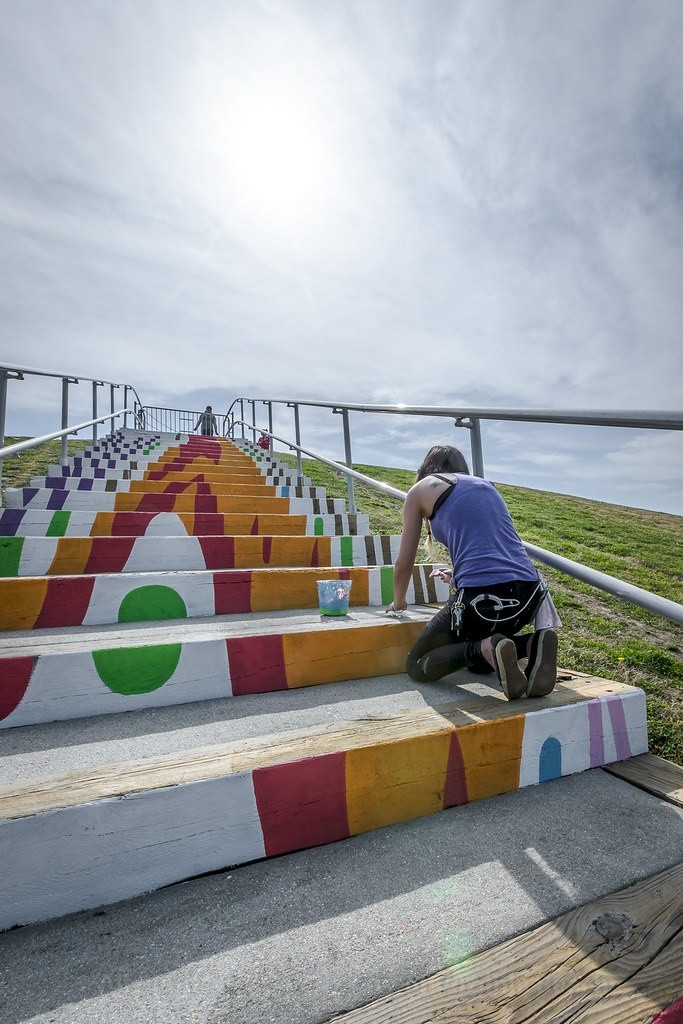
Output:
[317,580,352,616]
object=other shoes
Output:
[526,627,558,697]
[491,633,528,700]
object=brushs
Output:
[429,569,452,578]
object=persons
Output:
[386,446,558,698]
[258,429,270,449]
[193,406,218,435]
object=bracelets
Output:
[393,603,407,613]
